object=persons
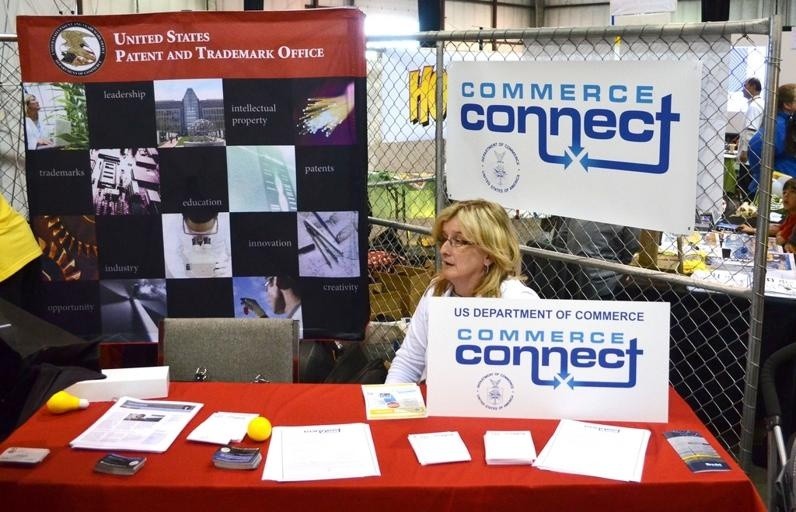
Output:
[555,213,642,299]
[737,79,766,197]
[241,276,303,337]
[0,193,44,325]
[24,95,50,150]
[740,177,796,244]
[748,82,796,198]
[382,195,549,390]
[163,212,234,277]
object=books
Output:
[361,380,429,422]
[662,429,732,473]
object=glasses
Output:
[441,234,473,249]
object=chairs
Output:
[156,315,299,383]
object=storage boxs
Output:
[366,262,429,321]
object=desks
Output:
[0,379,769,510]
